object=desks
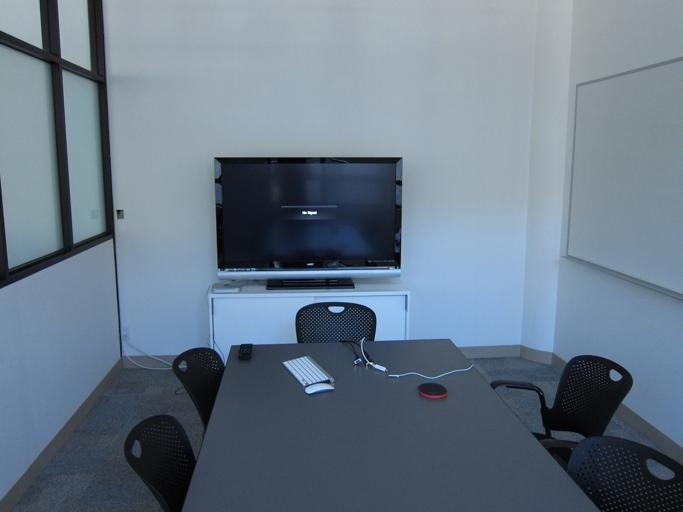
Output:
[181,339,597,511]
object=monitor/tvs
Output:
[213,156,404,290]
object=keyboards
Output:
[281,354,335,386]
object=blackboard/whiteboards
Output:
[566,56,683,302]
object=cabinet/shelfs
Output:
[209,299,411,358]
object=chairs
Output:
[296,303,377,341]
[122,416,194,510]
[568,438,683,511]
[491,354,634,460]
[172,346,225,429]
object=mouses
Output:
[305,383,336,395]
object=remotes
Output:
[238,344,252,361]
